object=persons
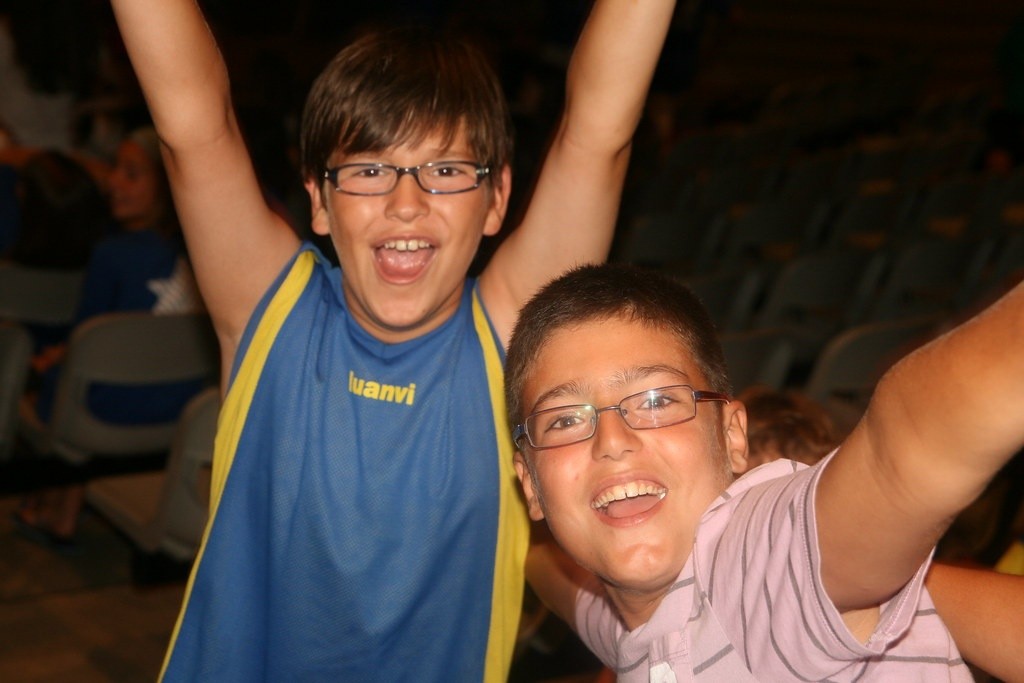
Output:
[506,262,1024,683]
[0,12,321,549]
[105,0,682,682]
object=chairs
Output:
[620,70,1024,438]
[0,258,225,562]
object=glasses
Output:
[513,384,731,450]
[318,161,490,196]
